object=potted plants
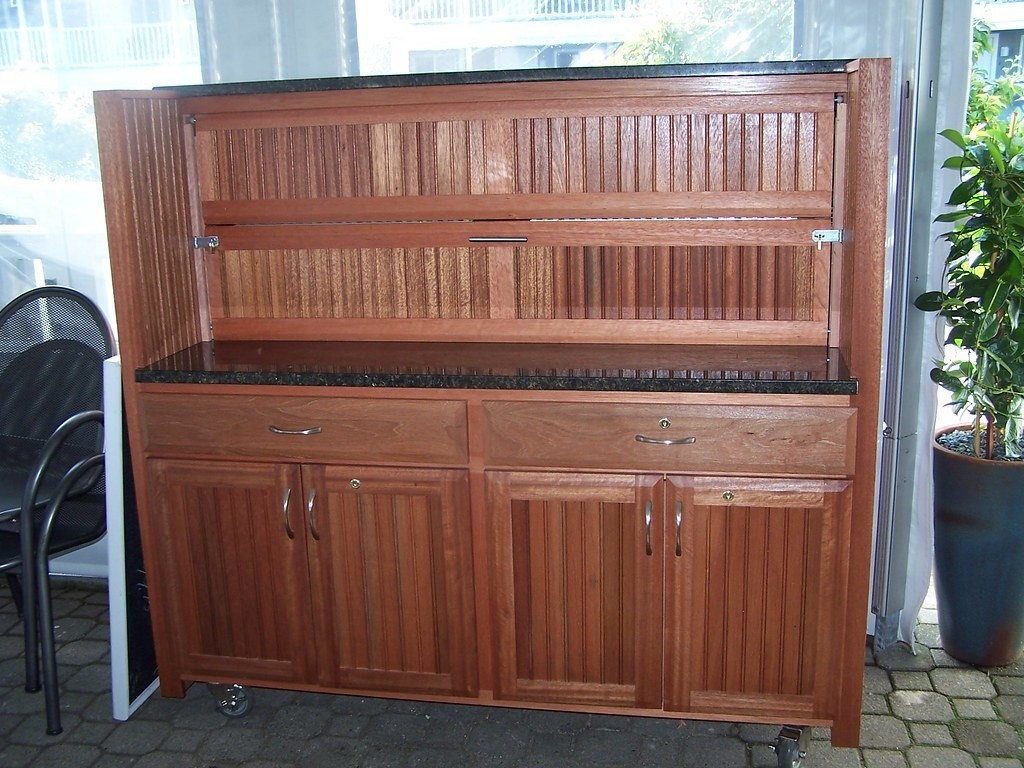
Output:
[913,110,1024,670]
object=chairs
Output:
[0,285,117,738]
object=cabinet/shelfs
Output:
[92,56,895,768]
